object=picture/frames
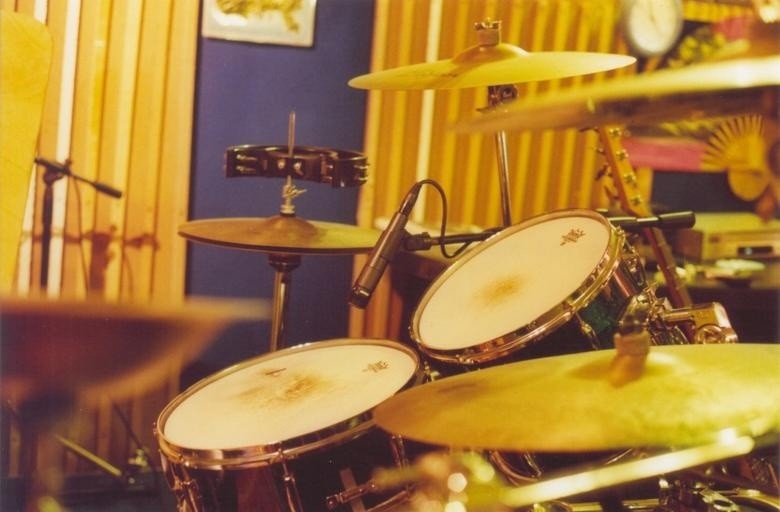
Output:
[200,0,318,49]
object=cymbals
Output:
[451,56,775,131]
[348,44,636,91]
[373,341,776,453]
[179,216,383,256]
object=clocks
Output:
[620,0,685,57]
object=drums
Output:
[151,338,441,510]
[410,208,691,503]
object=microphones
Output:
[84,178,124,198]
[350,184,417,310]
